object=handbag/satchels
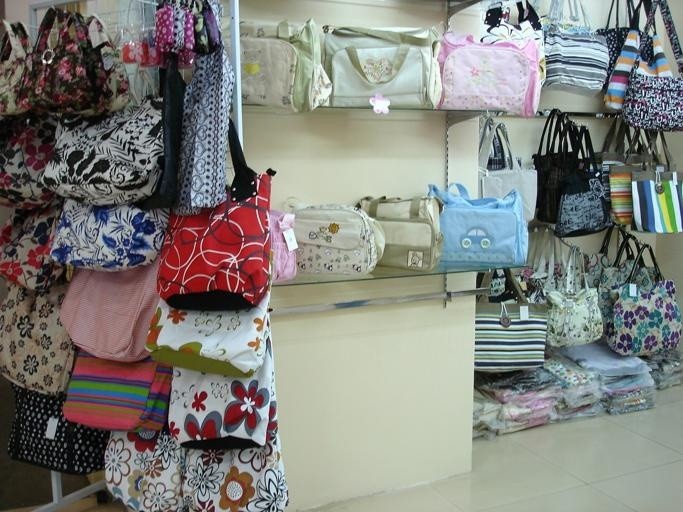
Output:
[475,252,682,376]
[0,0,296,512]
[358,194,441,272]
[293,204,386,279]
[323,23,442,111]
[480,153,683,238]
[235,19,333,114]
[429,183,531,271]
[438,18,683,129]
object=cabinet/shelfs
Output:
[232,0,487,511]
